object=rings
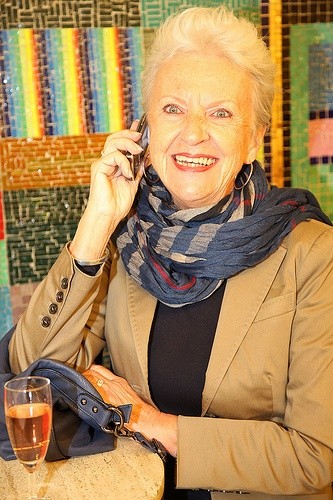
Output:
[96,379,105,387]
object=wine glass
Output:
[4,377,52,500]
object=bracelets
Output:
[75,247,111,266]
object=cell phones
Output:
[126,113,149,181]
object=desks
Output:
[0,434,165,500]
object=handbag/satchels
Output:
[1,357,170,463]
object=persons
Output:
[7,5,333,500]
